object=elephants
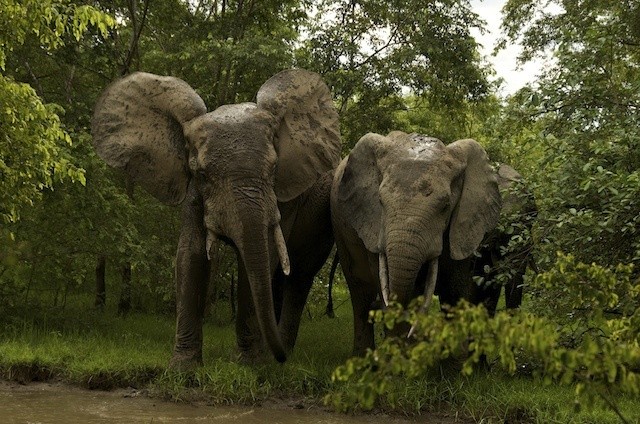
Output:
[319,130,503,374]
[433,163,540,326]
[90,68,343,389]
